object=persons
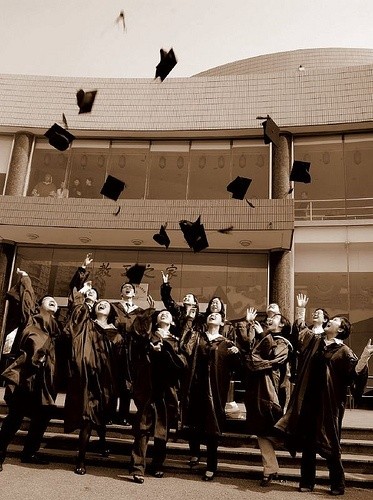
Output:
[67,253,330,429]
[0,268,69,472]
[244,308,293,486]
[274,294,373,494]
[32,173,100,199]
[128,295,188,484]
[295,191,310,220]
[180,308,243,481]
[63,281,131,475]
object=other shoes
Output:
[203,470,216,481]
[21,453,51,465]
[153,470,164,478]
[330,488,345,496]
[189,457,200,465]
[76,460,86,475]
[134,475,144,483]
[261,471,278,487]
[298,486,315,492]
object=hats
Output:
[101,174,126,216]
[256,114,280,148]
[124,263,146,286]
[152,221,171,250]
[43,112,76,152]
[76,88,98,115]
[226,175,255,208]
[217,226,233,235]
[287,160,312,195]
[153,47,178,84]
[116,10,125,29]
[178,215,209,255]
